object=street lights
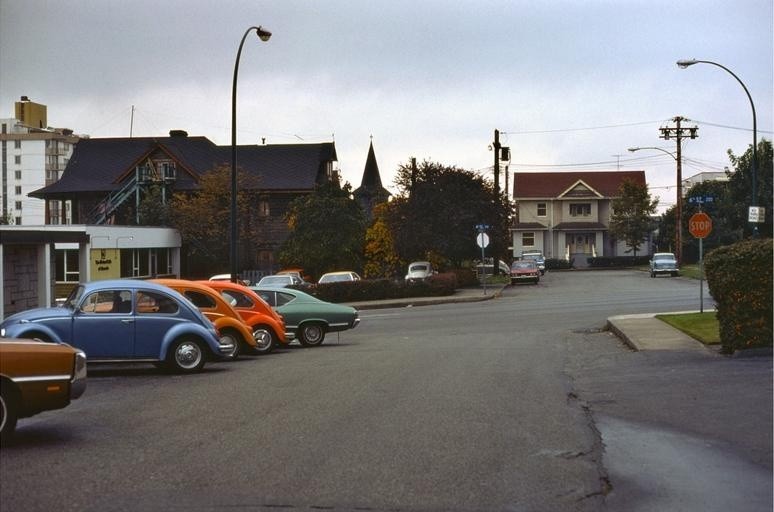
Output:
[676,59,760,238]
[628,148,678,160]
[230,26,271,283]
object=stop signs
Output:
[689,213,713,240]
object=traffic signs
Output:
[474,224,492,229]
[688,195,716,204]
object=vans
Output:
[521,253,545,276]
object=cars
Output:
[1,274,286,376]
[0,338,87,445]
[649,252,680,277]
[404,261,434,286]
[246,285,360,346]
[510,260,540,283]
[476,266,509,279]
[257,268,313,287]
[318,272,362,284]
